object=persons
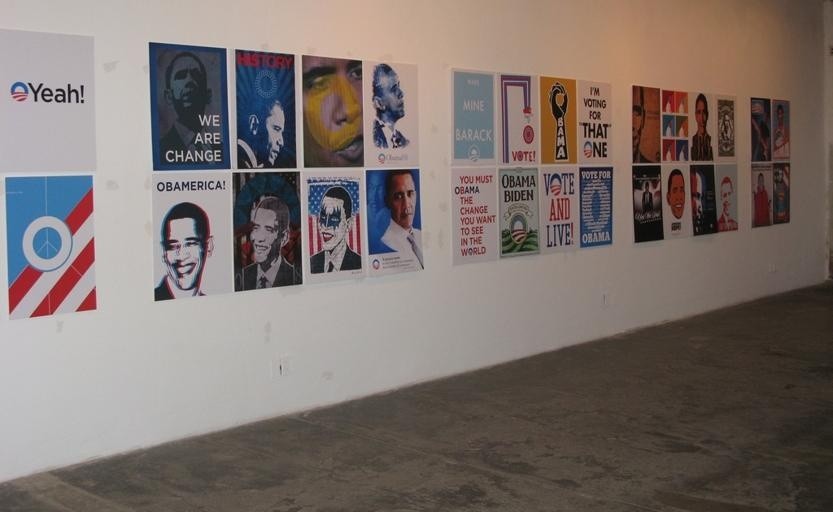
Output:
[642,181,653,212]
[665,120,673,137]
[378,171,423,270]
[665,97,673,113]
[679,144,686,160]
[692,93,713,161]
[367,171,384,213]
[753,172,771,225]
[154,202,214,300]
[160,53,222,162]
[237,96,286,167]
[632,86,652,164]
[666,169,685,219]
[721,113,732,147]
[717,177,738,232]
[774,168,790,220]
[679,118,686,138]
[752,101,770,161]
[301,55,363,167]
[370,64,408,149]
[309,186,362,274]
[774,105,789,157]
[690,167,704,234]
[234,196,302,291]
[665,143,672,160]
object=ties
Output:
[407,234,423,269]
